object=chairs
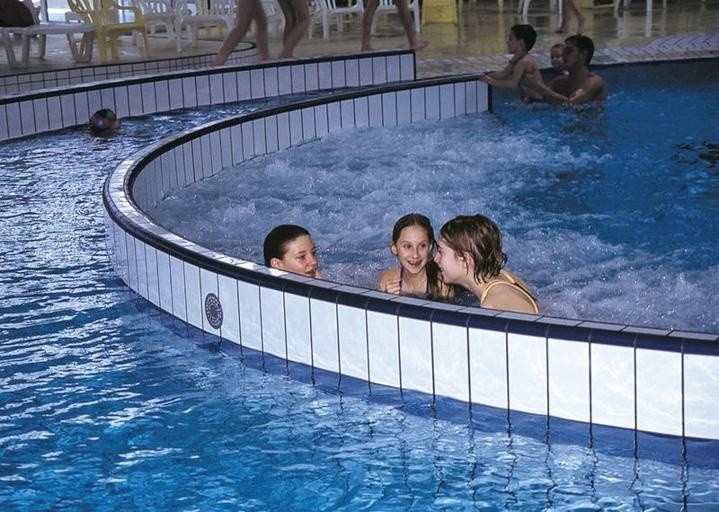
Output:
[0,0,661,69]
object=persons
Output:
[88,106,121,133]
[521,35,611,100]
[275,0,313,59]
[549,43,569,77]
[554,0,586,35]
[210,0,272,67]
[480,24,545,105]
[432,212,540,314]
[262,224,320,278]
[359,2,430,52]
[378,212,455,301]
